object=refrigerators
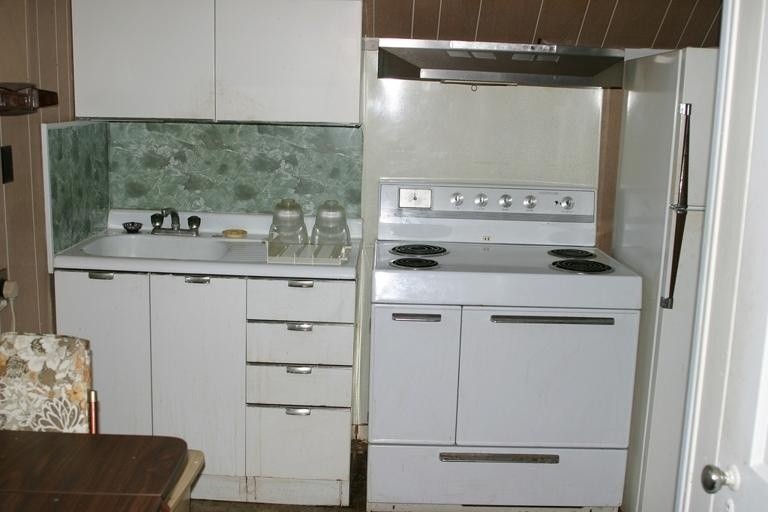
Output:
[605,47,724,510]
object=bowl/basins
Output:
[122,222,142,233]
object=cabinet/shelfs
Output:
[146,267,251,505]
[247,267,361,510]
[49,267,154,438]
[67,0,365,130]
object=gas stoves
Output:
[368,238,642,312]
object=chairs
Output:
[0,330,99,434]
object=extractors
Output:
[378,34,625,95]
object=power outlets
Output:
[0,265,11,312]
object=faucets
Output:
[162,207,181,229]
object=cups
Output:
[271,201,309,245]
[310,199,353,245]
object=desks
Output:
[0,427,209,512]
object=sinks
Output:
[83,235,229,261]
